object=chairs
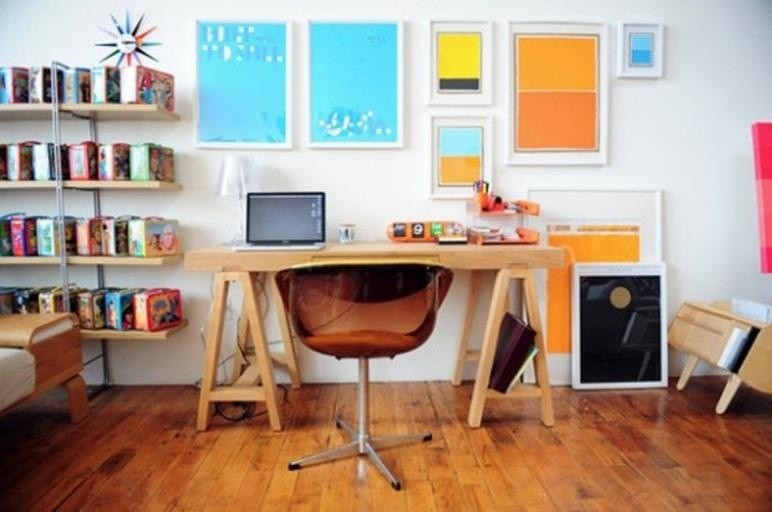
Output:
[273,261,456,491]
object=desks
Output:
[183,239,569,431]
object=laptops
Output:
[232,189,326,250]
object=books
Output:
[474,226,520,243]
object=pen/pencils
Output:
[473,180,491,213]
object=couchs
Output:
[0,312,93,422]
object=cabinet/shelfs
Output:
[0,61,190,402]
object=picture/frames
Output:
[430,20,496,108]
[617,21,666,80]
[571,259,670,392]
[426,116,494,203]
[504,18,611,167]
[302,15,407,151]
[519,187,667,389]
[191,15,292,150]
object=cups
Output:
[337,225,356,244]
